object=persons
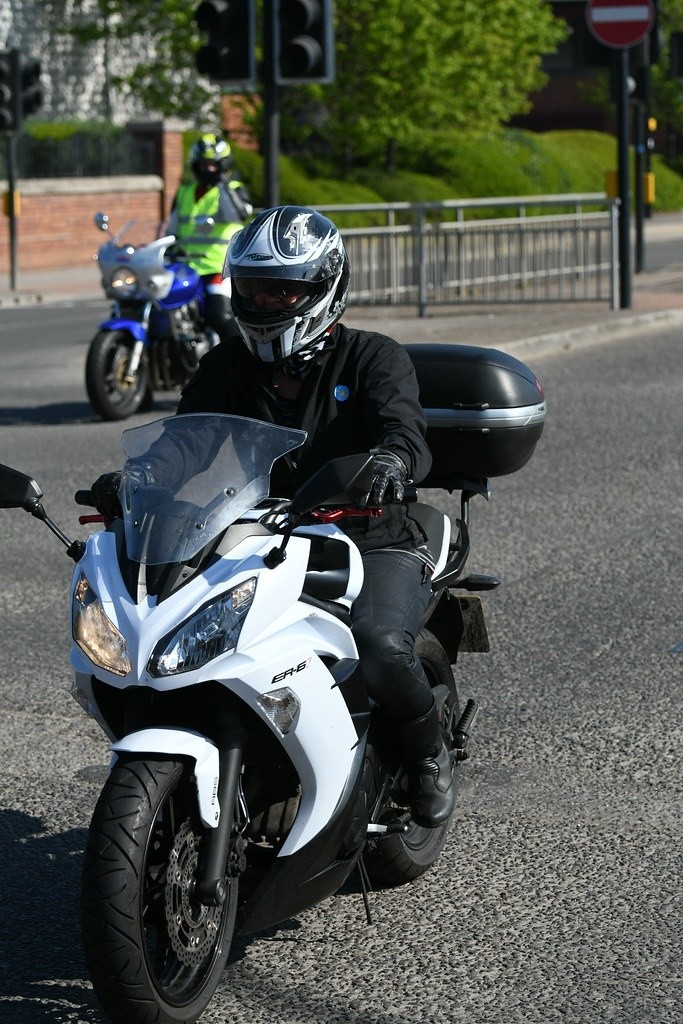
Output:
[167,133,254,342]
[90,205,456,827]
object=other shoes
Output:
[134,395,156,414]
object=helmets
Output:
[228,206,350,364]
[191,133,233,186]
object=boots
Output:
[388,692,457,829]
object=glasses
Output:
[241,280,317,299]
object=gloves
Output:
[90,460,155,526]
[348,452,407,507]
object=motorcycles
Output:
[0,346,548,1022]
[83,210,237,422]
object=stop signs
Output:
[584,0,657,50]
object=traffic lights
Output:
[607,45,650,104]
[17,52,45,120]
[267,0,339,87]
[0,50,21,133]
[193,0,256,87]
